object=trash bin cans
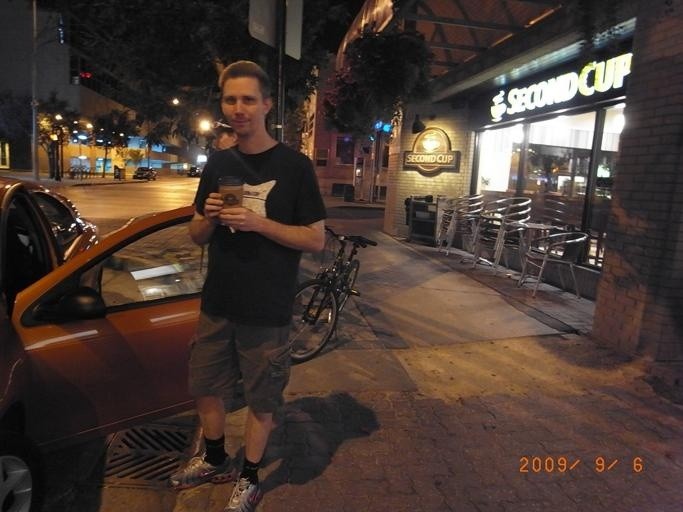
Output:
[343,184,354,202]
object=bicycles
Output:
[288,224,379,363]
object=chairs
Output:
[404,194,591,299]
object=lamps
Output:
[411,111,436,134]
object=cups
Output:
[215,176,244,226]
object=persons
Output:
[165,62,328,512]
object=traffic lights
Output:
[78,70,96,80]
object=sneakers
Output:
[224,478,263,511]
[168,453,237,489]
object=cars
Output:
[131,166,157,181]
[185,166,202,177]
[0,173,211,511]
